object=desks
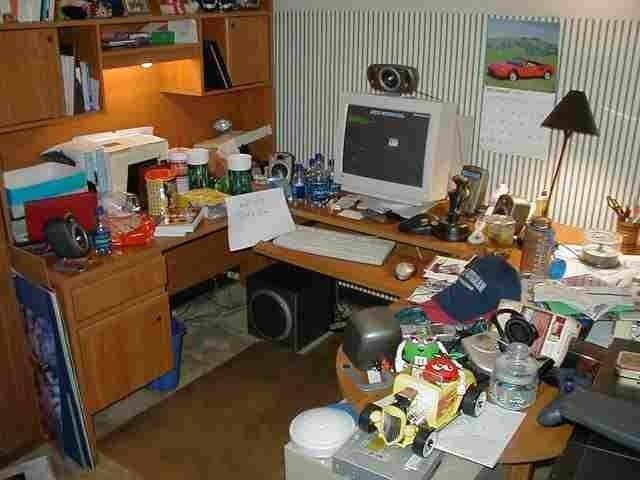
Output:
[162,197,520,312]
[334,218,586,464]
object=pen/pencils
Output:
[622,206,640,224]
[560,241,584,261]
[415,246,423,261]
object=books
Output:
[61,54,101,116]
[421,253,468,285]
[155,204,202,237]
[204,40,231,93]
[0,0,55,25]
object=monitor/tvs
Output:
[332,92,473,219]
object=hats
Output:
[431,254,522,320]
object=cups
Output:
[482,213,517,262]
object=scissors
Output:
[607,196,624,215]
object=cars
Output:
[487,57,556,80]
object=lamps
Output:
[537,87,599,223]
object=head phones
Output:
[492,307,555,379]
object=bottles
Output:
[521,213,557,278]
[186,146,213,192]
[487,341,541,413]
[89,205,113,257]
[225,154,255,197]
[288,151,341,212]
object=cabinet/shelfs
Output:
[161,9,273,99]
[97,16,200,69]
[47,249,173,463]
[0,20,101,136]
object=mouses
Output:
[395,262,416,280]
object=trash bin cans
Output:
[145,318,188,392]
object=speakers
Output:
[461,165,489,215]
[44,217,89,272]
[342,306,400,392]
[493,194,531,238]
[270,151,295,183]
[245,263,336,351]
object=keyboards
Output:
[273,223,395,266]
